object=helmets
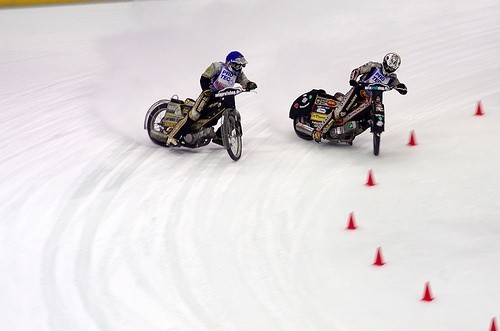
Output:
[383,53,401,76]
[226,51,247,74]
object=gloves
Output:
[350,79,357,87]
[397,83,405,92]
[246,82,257,90]
[203,88,212,96]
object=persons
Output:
[311,52,407,144]
[165,51,257,149]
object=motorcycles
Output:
[143,87,242,160]
[289,80,406,155]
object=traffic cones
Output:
[405,130,417,146]
[346,212,357,229]
[473,100,485,116]
[365,170,377,185]
[489,318,498,331]
[372,247,386,266]
[421,281,435,301]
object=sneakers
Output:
[212,137,232,146]
[167,138,177,146]
[312,131,321,144]
